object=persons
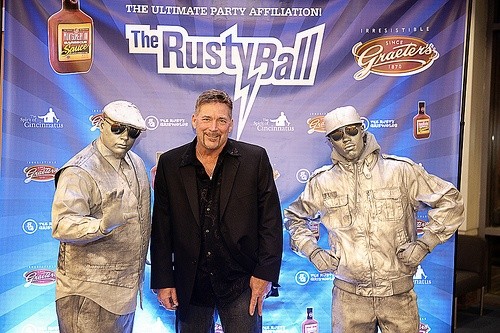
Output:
[284,105,466,333]
[50,100,152,333]
[150,90,283,333]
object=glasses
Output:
[103,118,142,139]
[330,125,361,141]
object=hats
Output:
[323,106,362,136]
[104,101,146,131]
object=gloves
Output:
[396,241,430,268]
[310,248,340,272]
[99,188,137,234]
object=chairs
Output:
[453,235,489,331]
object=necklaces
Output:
[197,150,217,180]
